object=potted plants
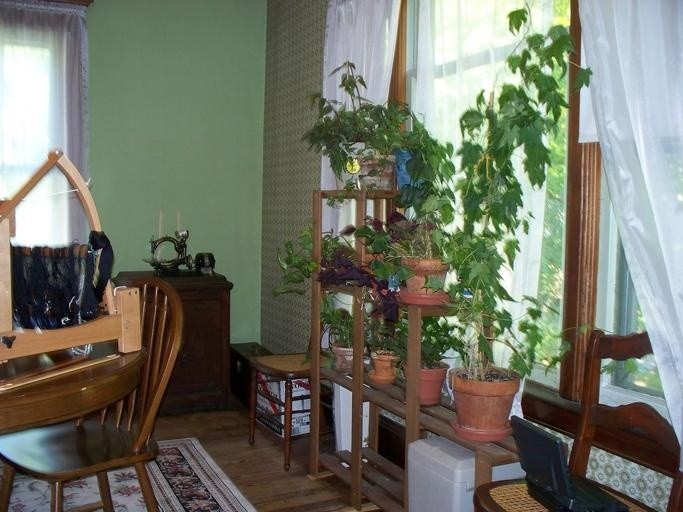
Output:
[269,0,590,443]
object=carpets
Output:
[0,440,260,510]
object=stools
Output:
[249,354,334,470]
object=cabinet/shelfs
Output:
[111,269,234,417]
[313,190,519,512]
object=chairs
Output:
[472,331,683,510]
[0,276,185,510]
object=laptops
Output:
[511,415,630,512]
